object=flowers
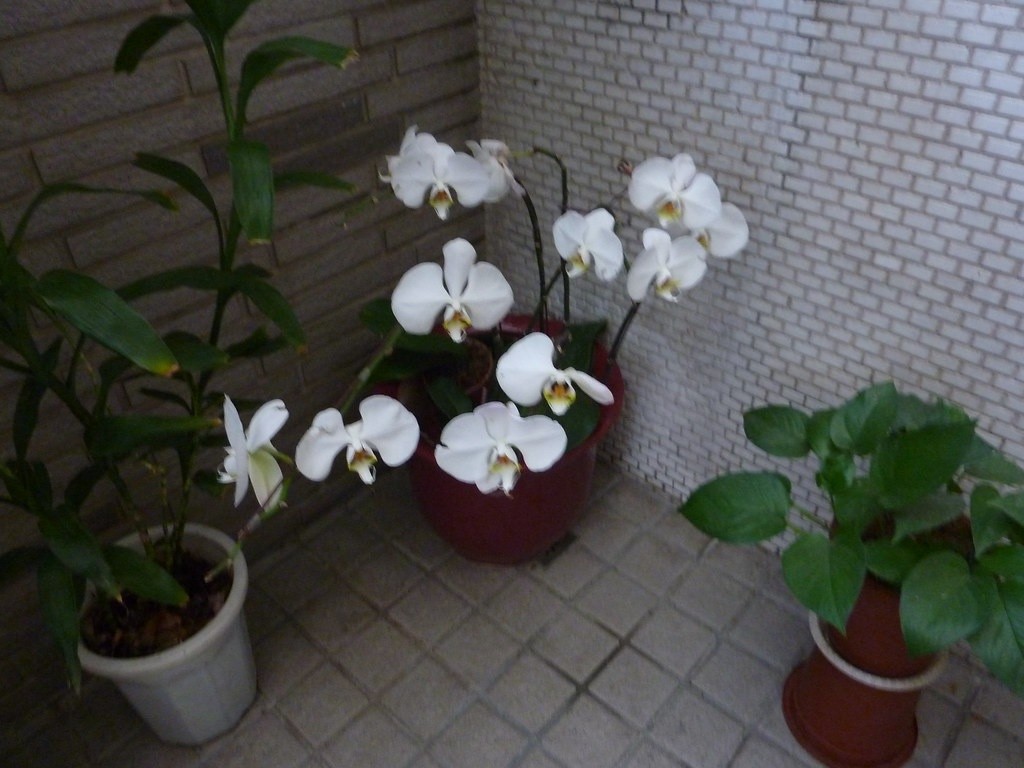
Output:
[218,123,749,508]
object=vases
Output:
[369,312,619,564]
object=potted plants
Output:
[0,0,360,747]
[678,380,1024,768]
